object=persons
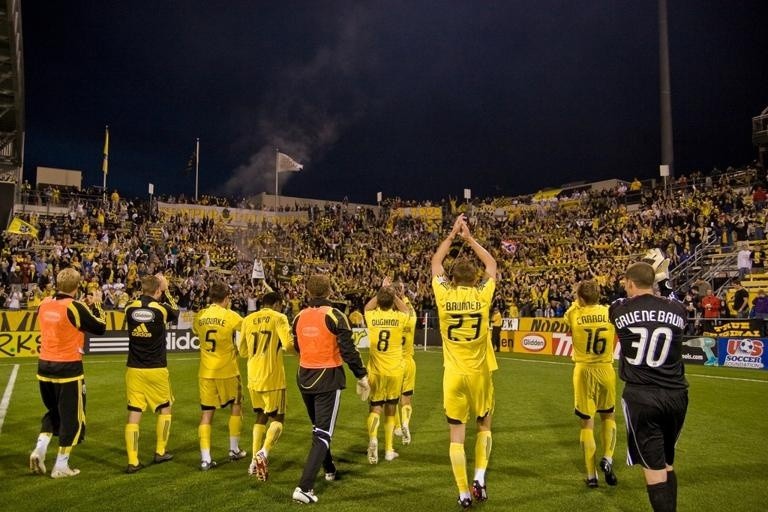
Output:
[291,275,371,503]
[20,179,32,205]
[29,268,107,480]
[499,195,557,318]
[433,194,497,213]
[238,291,294,483]
[40,183,78,302]
[691,285,703,317]
[751,289,768,319]
[701,289,720,318]
[558,165,665,312]
[79,184,290,308]
[669,164,768,274]
[125,273,180,473]
[0,239,39,311]
[363,276,409,463]
[391,281,418,446]
[725,280,749,318]
[429,213,498,507]
[608,248,690,512]
[491,307,503,353]
[691,277,712,297]
[288,196,429,302]
[190,286,244,471]
[562,281,619,489]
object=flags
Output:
[7,218,39,237]
[276,153,304,173]
[102,130,109,174]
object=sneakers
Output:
[456,495,472,510]
[293,487,319,504]
[256,451,269,482]
[384,451,399,461]
[324,469,337,482]
[154,454,174,463]
[127,463,145,473]
[367,439,379,465]
[200,461,218,471]
[599,459,617,486]
[402,422,411,445]
[584,477,597,487]
[472,479,488,502]
[29,455,47,475]
[49,467,81,478]
[228,450,247,461]
[248,461,257,479]
[394,429,402,437]
